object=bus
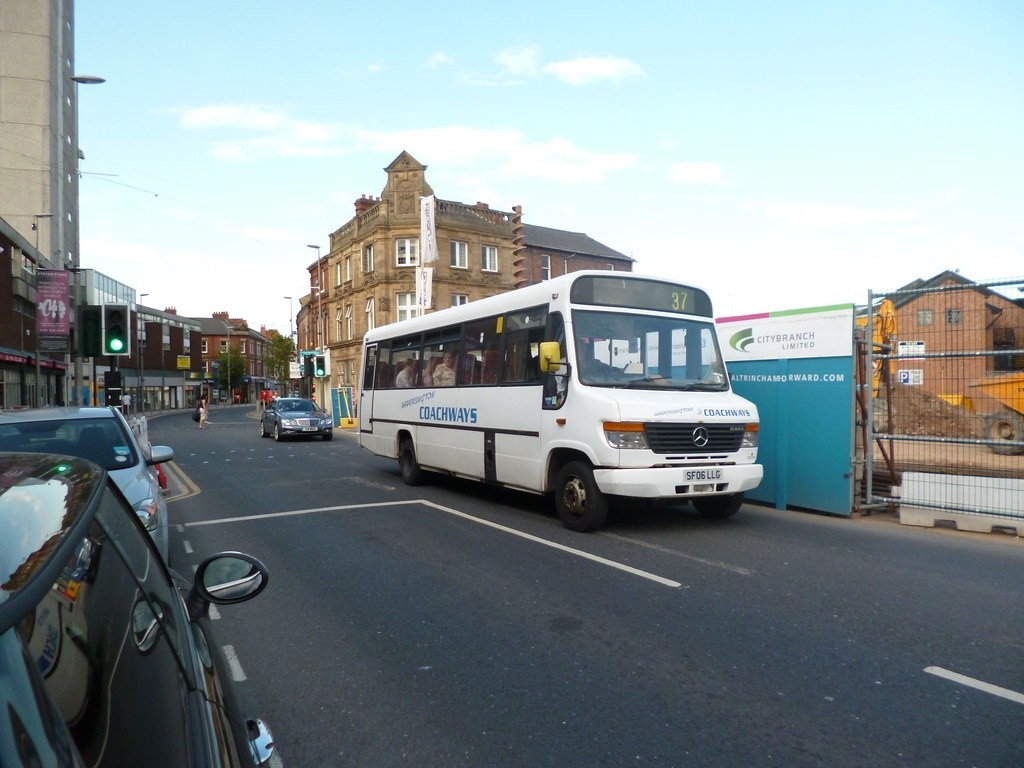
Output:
[356,269,765,533]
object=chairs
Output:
[375,332,532,389]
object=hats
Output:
[273,391,278,395]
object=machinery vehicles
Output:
[937,370,1024,456]
[853,299,898,432]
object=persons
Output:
[122,393,131,414]
[198,394,208,429]
[433,350,456,388]
[260,388,278,406]
[396,358,414,388]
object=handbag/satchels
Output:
[192,407,200,422]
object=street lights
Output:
[139,293,149,415]
[33,211,55,407]
[307,244,326,411]
[226,326,235,406]
[284,296,295,398]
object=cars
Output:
[259,395,333,443]
[0,449,278,768]
[0,403,177,576]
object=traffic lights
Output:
[101,303,131,357]
[315,355,326,377]
